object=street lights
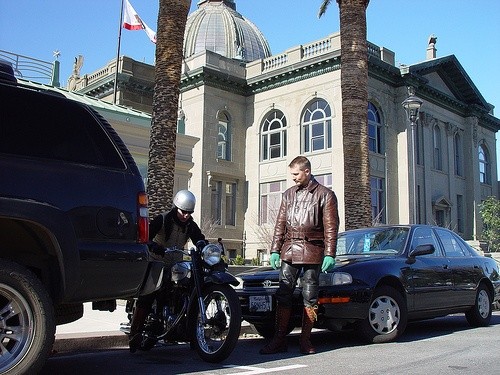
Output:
[401,85,424,224]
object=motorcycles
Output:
[120,238,244,363]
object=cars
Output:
[229,223,499,343]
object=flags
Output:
[121,0,157,43]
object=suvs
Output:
[0,73,151,370]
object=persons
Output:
[129,189,226,351]
[259,156,339,354]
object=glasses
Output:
[180,209,193,214]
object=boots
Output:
[129,306,149,353]
[260,306,290,354]
[190,338,213,350]
[300,307,318,354]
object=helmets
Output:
[173,190,195,211]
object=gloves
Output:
[270,253,280,270]
[321,256,335,273]
[152,242,165,258]
